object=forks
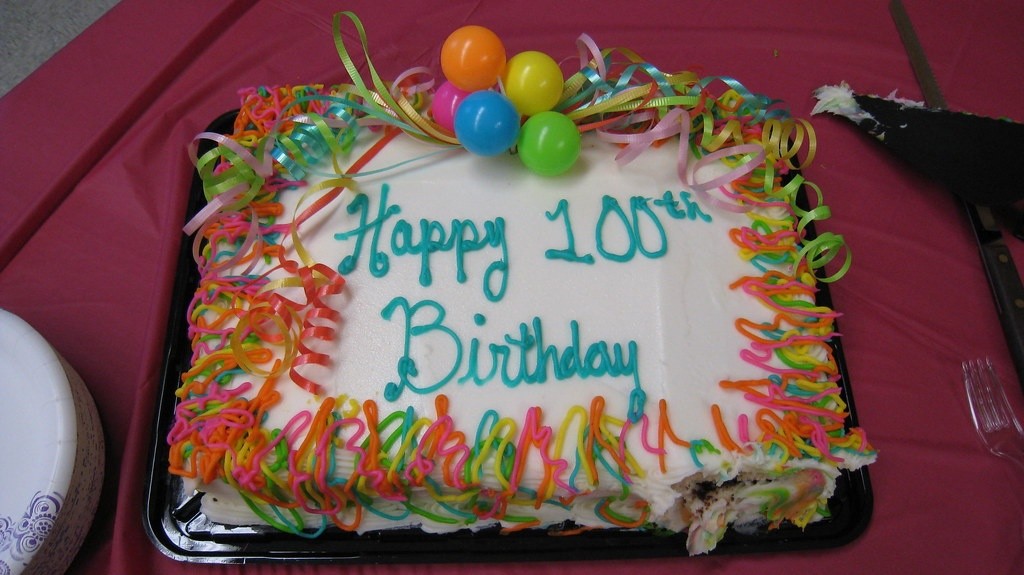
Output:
[960,355,1024,469]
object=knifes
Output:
[889,0,1024,391]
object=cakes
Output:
[166,9,879,558]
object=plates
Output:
[0,306,105,575]
[142,107,875,567]
